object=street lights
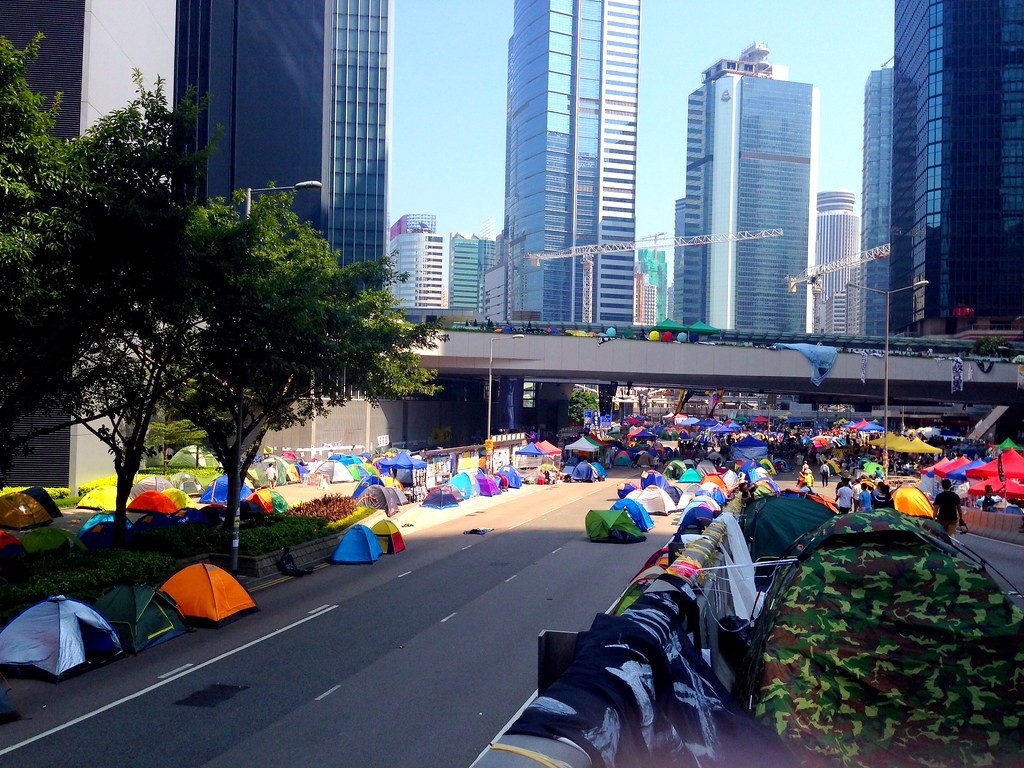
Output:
[846,280,931,478]
[229,179,322,572]
[487,334,525,473]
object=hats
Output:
[805,470,809,474]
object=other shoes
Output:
[823,486,824,487]
[826,484,828,486]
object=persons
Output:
[819,461,831,487]
[801,461,810,474]
[871,484,894,510]
[932,479,964,544]
[857,483,873,511]
[981,483,1007,513]
[265,463,277,489]
[834,478,854,512]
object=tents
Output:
[449,472,480,500]
[648,318,689,343]
[421,488,459,510]
[314,460,355,484]
[161,560,260,633]
[688,321,723,344]
[198,474,253,504]
[1,412,1024,768]
[127,491,177,516]
[76,486,131,512]
[372,519,406,554]
[332,525,383,564]
[94,580,193,655]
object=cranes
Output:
[641,233,669,260]
[525,229,783,323]
[787,243,891,333]
